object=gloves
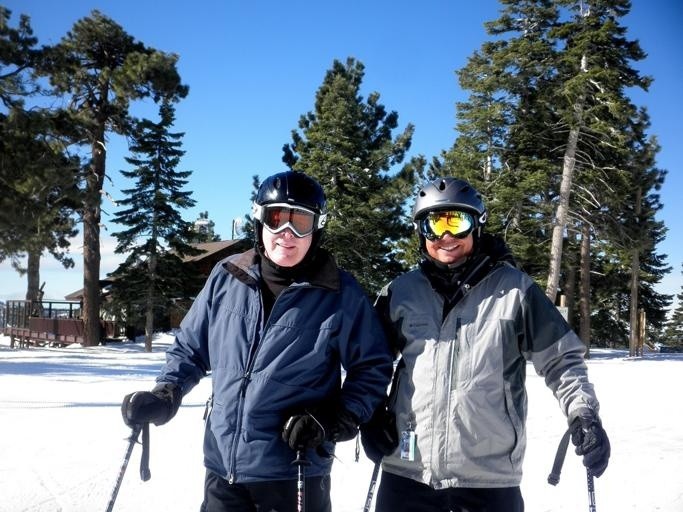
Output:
[120,383,181,428]
[359,402,399,464]
[281,402,359,451]
[571,415,610,478]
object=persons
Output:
[358,176,610,511]
[120,171,392,511]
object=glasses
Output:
[252,201,327,238]
[417,209,479,240]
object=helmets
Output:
[253,170,325,215]
[413,177,486,224]
